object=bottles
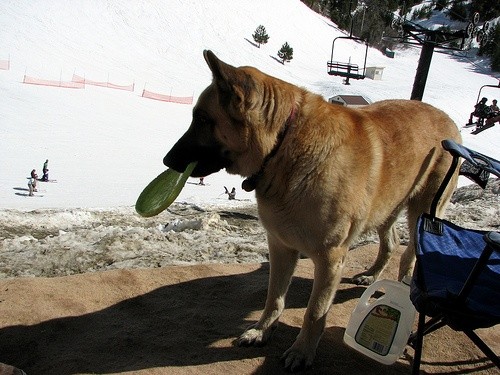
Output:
[343,273,417,366]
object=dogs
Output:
[163,50,464,372]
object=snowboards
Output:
[471,123,494,135]
[462,120,482,129]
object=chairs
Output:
[408,139,500,375]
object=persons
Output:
[469,97,488,127]
[27,176,37,197]
[228,187,236,200]
[42,159,49,181]
[485,99,500,125]
[31,168,38,179]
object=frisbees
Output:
[133,161,194,217]
